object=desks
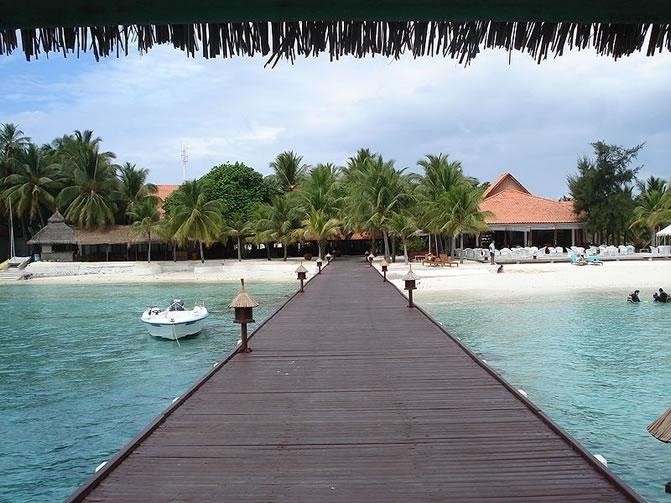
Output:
[415,255,425,261]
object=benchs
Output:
[639,249,649,253]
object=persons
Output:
[489,239,497,265]
[579,254,588,266]
[627,289,641,303]
[653,287,669,303]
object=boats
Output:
[140,297,211,341]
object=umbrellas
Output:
[657,224,671,237]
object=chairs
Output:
[649,245,671,256]
[455,244,636,266]
[422,254,458,267]
